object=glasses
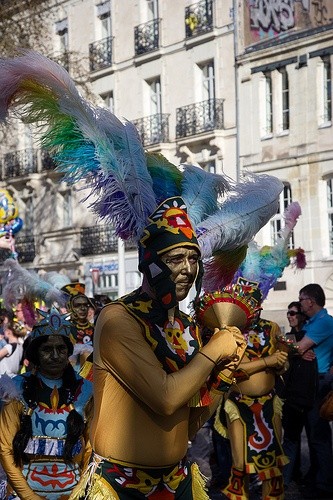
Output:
[287,311,298,316]
[16,310,23,312]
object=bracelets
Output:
[263,358,269,368]
[198,351,218,367]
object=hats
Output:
[89,294,112,315]
[30,303,75,341]
[239,278,262,301]
[60,283,96,319]
[0,298,17,318]
[138,196,202,274]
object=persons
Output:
[0,198,333,500]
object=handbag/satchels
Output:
[276,348,320,400]
